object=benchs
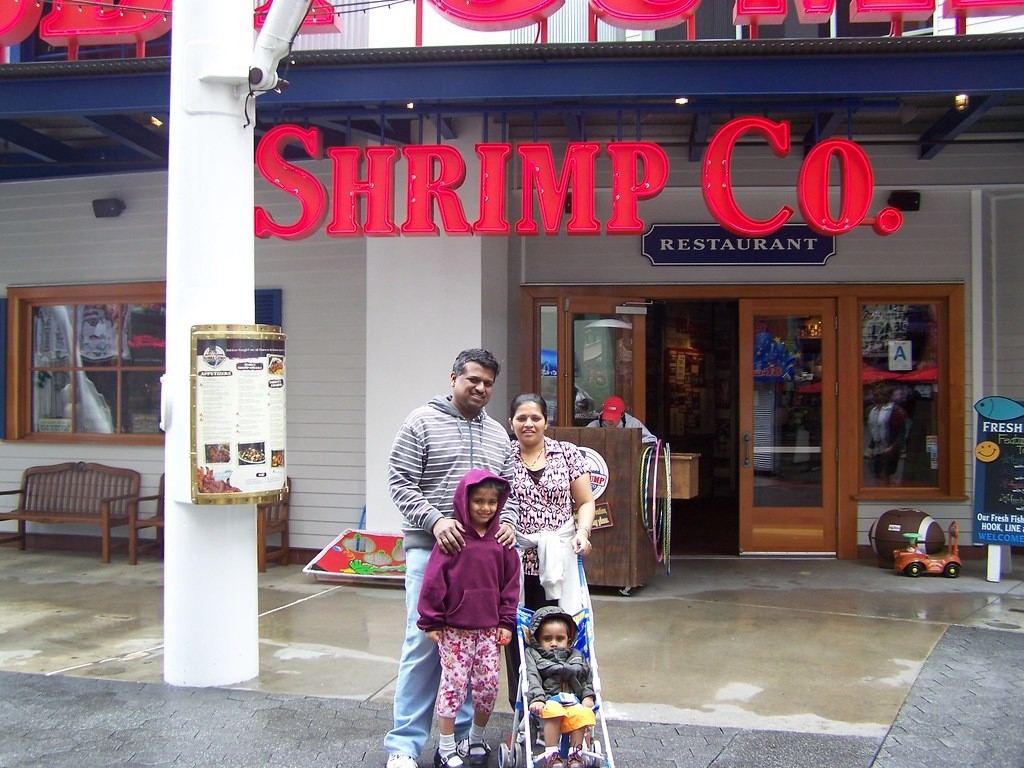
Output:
[126,469,293,573]
[0,461,140,562]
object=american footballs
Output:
[867,508,946,565]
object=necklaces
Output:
[519,447,545,469]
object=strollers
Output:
[496,530,616,768]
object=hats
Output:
[603,396,626,422]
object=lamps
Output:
[887,191,921,213]
[91,198,126,219]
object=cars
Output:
[892,519,963,580]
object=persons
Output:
[862,382,906,487]
[383,349,595,768]
[585,396,657,444]
[521,605,595,768]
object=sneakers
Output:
[567,742,585,768]
[545,751,563,768]
[386,753,418,768]
[456,736,491,757]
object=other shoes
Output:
[434,748,465,768]
[468,742,488,768]
[515,731,526,744]
[537,735,564,750]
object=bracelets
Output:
[576,526,591,539]
